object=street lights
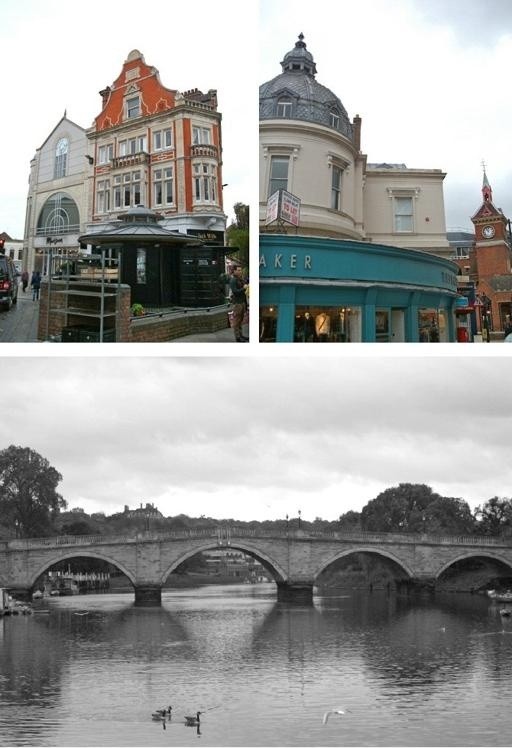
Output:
[297,509,301,527]
[398,519,404,529]
[14,518,20,539]
[420,511,426,533]
[145,510,151,531]
[479,292,492,341]
[285,513,291,527]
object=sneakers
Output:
[236,334,248,342]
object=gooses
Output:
[152,718,167,730]
[184,722,203,738]
[155,706,172,715]
[500,600,512,618]
[183,711,202,724]
[151,710,167,718]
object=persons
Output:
[31,271,41,301]
[21,268,28,292]
[229,265,249,342]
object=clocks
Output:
[482,225,496,239]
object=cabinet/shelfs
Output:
[47,249,122,343]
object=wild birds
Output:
[74,611,91,617]
[322,708,354,725]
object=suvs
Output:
[0,254,19,313]
